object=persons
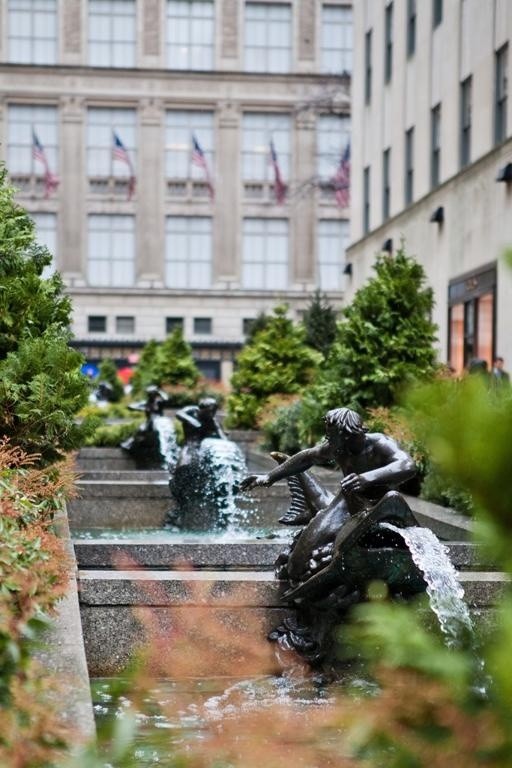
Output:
[491,357,504,376]
[128,386,169,444]
[241,408,417,586]
[176,397,225,468]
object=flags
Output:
[191,137,216,209]
[331,145,351,207]
[32,130,58,197]
[269,136,286,205]
[111,132,135,200]
